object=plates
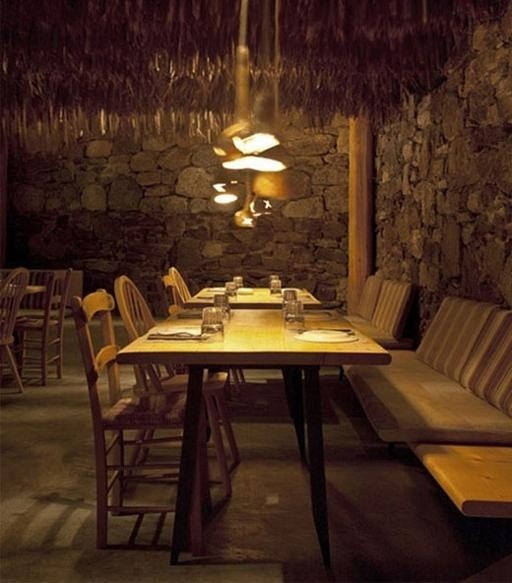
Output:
[295,329,359,343]
[158,326,200,336]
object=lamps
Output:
[211,0,289,226]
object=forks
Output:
[149,331,204,337]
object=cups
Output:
[283,301,305,330]
[283,290,298,313]
[233,276,242,289]
[270,280,283,298]
[225,282,237,302]
[270,275,278,282]
[213,294,231,324]
[200,307,224,342]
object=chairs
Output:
[12,268,73,386]
[168,266,246,400]
[155,276,240,411]
[116,276,241,497]
[0,268,29,395]
[24,270,54,313]
[71,288,203,556]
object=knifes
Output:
[148,335,209,341]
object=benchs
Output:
[342,275,415,349]
[342,294,512,518]
